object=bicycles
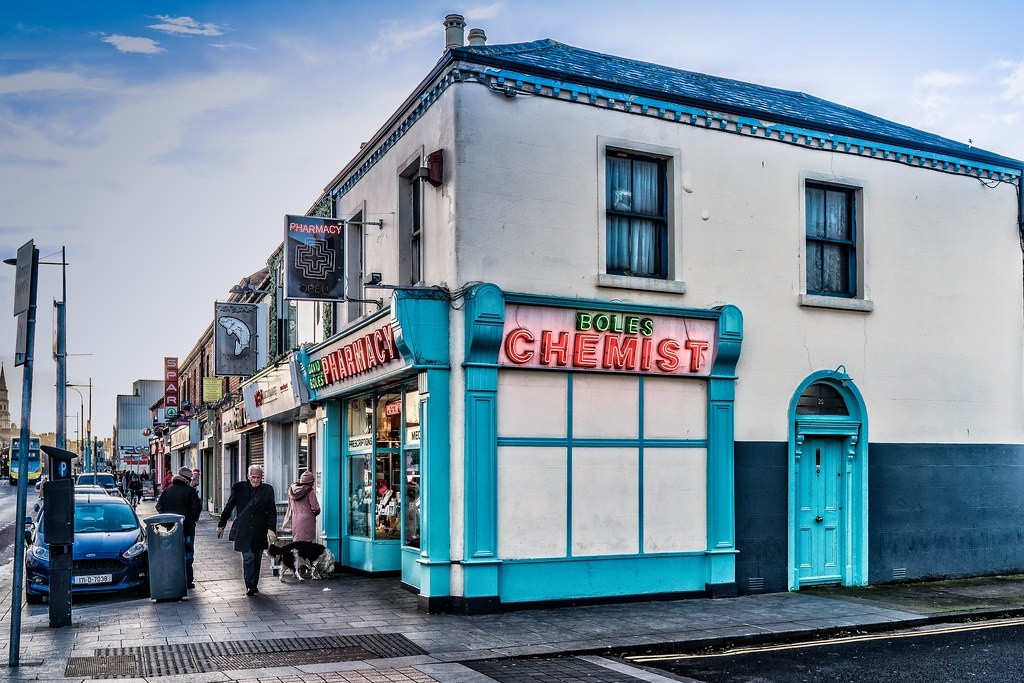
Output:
[127,489,143,513]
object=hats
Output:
[299,471,314,484]
[193,468,200,472]
[179,466,192,481]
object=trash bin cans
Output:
[142,514,191,604]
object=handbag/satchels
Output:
[282,505,292,532]
[229,518,238,541]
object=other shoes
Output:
[246,587,255,596]
[187,584,195,589]
[255,586,259,593]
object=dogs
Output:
[266,540,336,581]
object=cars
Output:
[23,496,151,604]
[34,472,124,512]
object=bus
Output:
[7,437,44,485]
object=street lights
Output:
[4,257,69,449]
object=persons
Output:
[190,469,201,494]
[288,471,321,578]
[106,456,117,474]
[216,464,278,596]
[157,466,202,589]
[113,470,155,505]
[161,470,174,496]
[38,477,48,500]
